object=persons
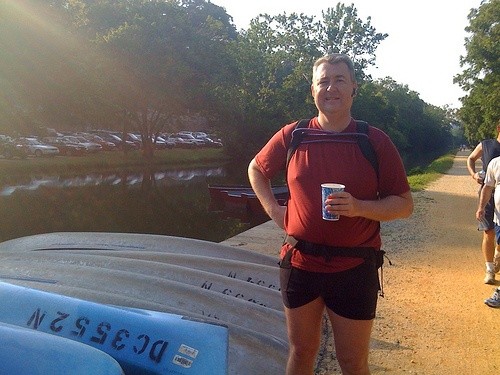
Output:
[248,54,414,375]
[467,130,500,284]
[476,156,500,308]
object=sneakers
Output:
[494,257,500,272]
[484,269,495,284]
[484,288,500,307]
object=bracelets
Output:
[473,175,475,179]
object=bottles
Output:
[478,169,486,178]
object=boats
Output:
[208,183,289,218]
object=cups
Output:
[321,184,346,221]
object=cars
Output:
[0,128,221,158]
